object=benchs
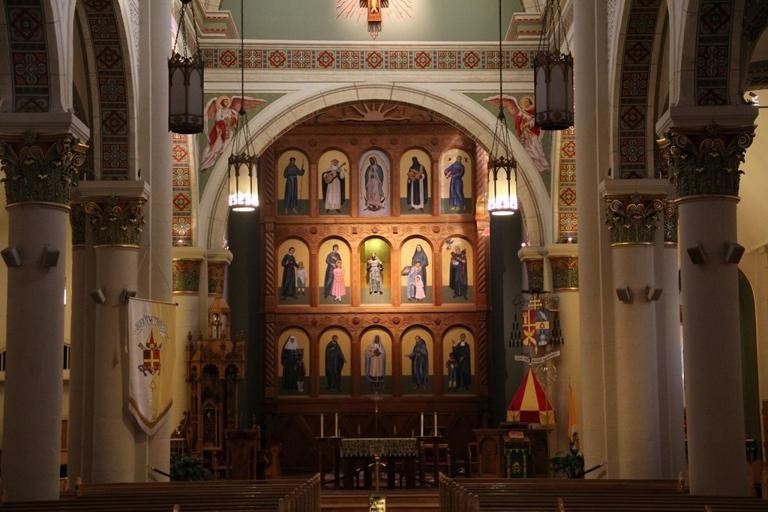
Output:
[436,472,766,512]
[0,473,323,512]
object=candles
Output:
[335,412,338,438]
[421,412,424,437]
[434,411,437,436]
[320,413,324,438]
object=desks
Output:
[311,434,442,490]
[472,427,551,478]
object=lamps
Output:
[89,287,137,304]
[532,0,574,130]
[167,0,204,134]
[486,0,520,216]
[615,285,663,303]
[227,0,258,214]
[1,246,60,267]
[687,243,745,265]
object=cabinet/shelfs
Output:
[226,427,259,480]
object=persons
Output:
[362,155,383,208]
[443,156,467,209]
[451,332,472,393]
[293,354,305,395]
[515,94,551,174]
[444,351,456,392]
[281,242,469,304]
[324,335,346,388]
[363,335,385,391]
[282,157,304,215]
[279,335,299,384]
[199,94,267,170]
[321,157,346,215]
[405,154,427,212]
[403,334,430,390]
